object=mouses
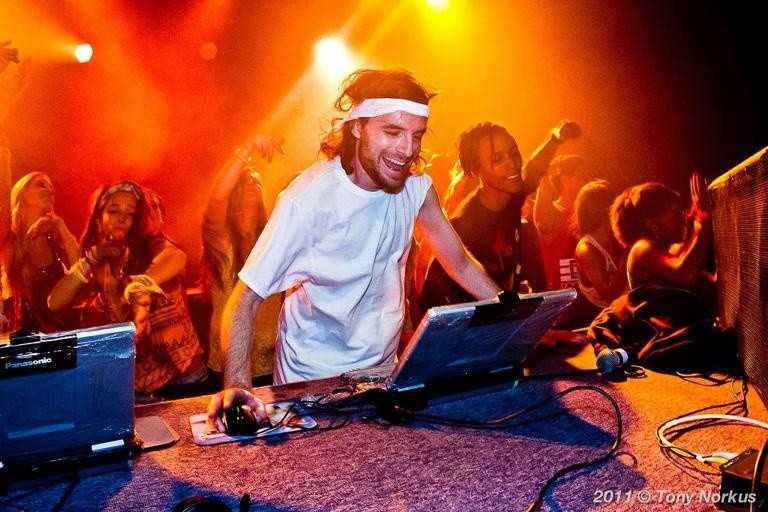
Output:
[219,399,259,435]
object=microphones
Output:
[595,345,637,374]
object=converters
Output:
[718,447,768,512]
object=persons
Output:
[143,189,165,235]
[606,174,716,290]
[199,134,286,394]
[396,119,626,357]
[0,132,82,337]
[48,180,210,402]
[207,66,589,436]
[0,40,19,74]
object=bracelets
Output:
[70,248,100,283]
[124,274,163,303]
[59,237,77,248]
[235,148,253,163]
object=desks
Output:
[0,345,767,512]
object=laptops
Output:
[0,321,139,484]
[339,288,578,405]
